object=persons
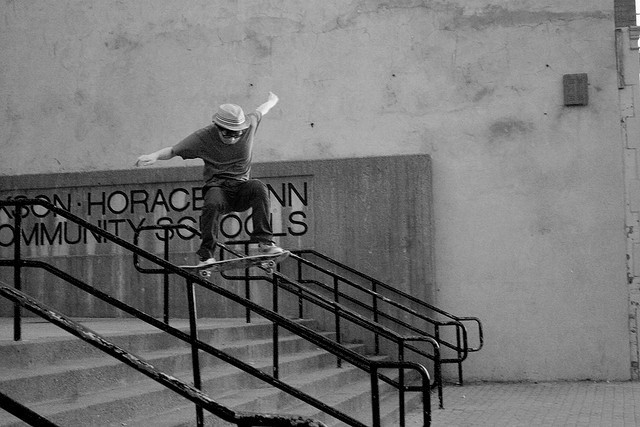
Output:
[133,90,284,264]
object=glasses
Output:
[219,129,248,139]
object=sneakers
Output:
[257,240,284,254]
[198,256,217,266]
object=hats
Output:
[212,103,252,131]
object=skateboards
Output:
[176,250,290,278]
